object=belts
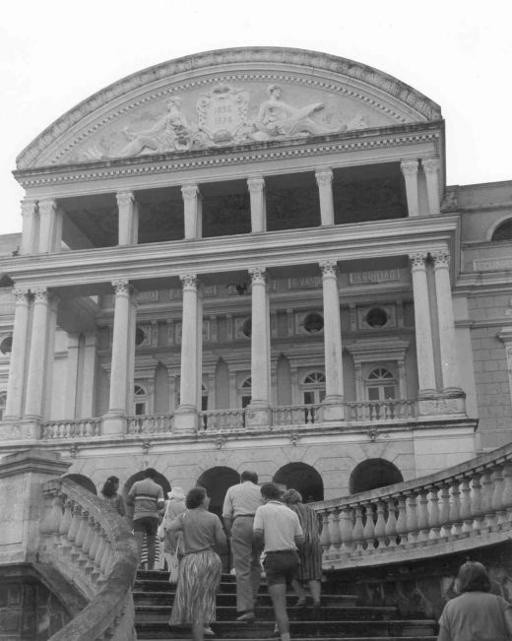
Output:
[237,514,255,517]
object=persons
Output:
[101,468,320,641]
[101,84,347,158]
[437,562,512,641]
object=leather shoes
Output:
[204,628,215,635]
[235,611,255,621]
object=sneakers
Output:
[270,630,291,641]
[294,598,307,607]
[312,601,320,605]
